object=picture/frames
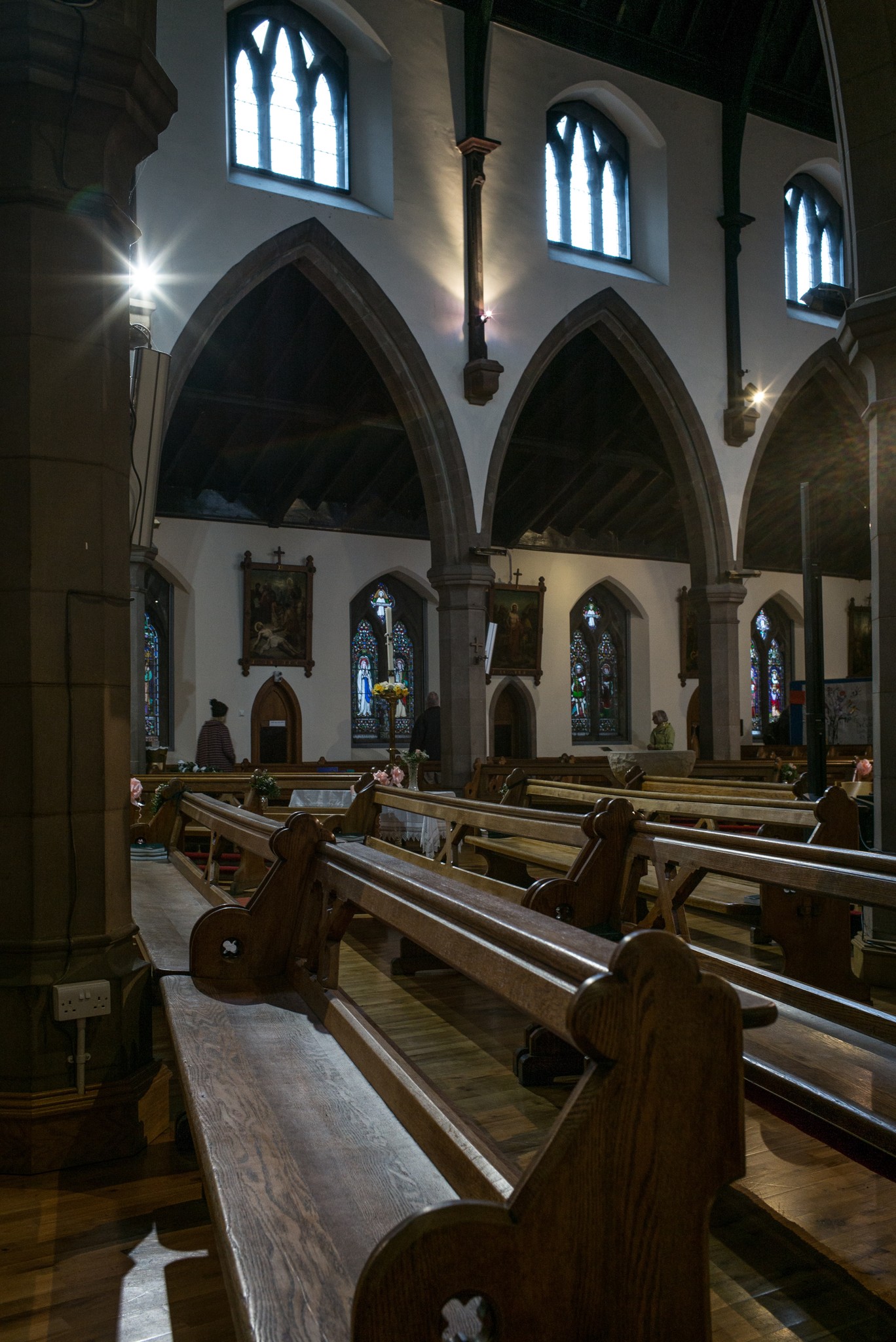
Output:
[676,585,704,688]
[238,545,316,678]
[845,594,872,680]
[485,568,547,687]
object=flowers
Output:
[175,758,219,773]
[149,784,166,811]
[371,680,411,699]
[780,762,798,781]
[369,764,407,789]
[250,768,281,796]
[399,742,430,763]
[856,756,872,776]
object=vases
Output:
[407,764,421,792]
[258,797,269,811]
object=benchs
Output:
[110,742,896,1342]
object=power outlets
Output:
[52,978,113,1022]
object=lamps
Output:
[744,381,760,404]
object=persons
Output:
[647,710,675,751]
[763,710,792,745]
[408,692,445,784]
[195,698,237,772]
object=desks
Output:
[289,789,457,857]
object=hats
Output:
[210,698,228,717]
